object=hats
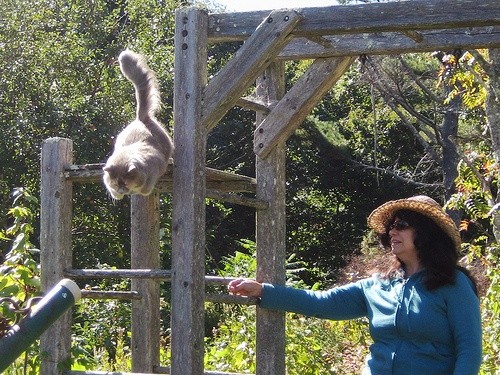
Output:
[365,195,462,254]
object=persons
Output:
[227,195,482,375]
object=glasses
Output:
[383,219,416,231]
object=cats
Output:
[102,50,174,200]
